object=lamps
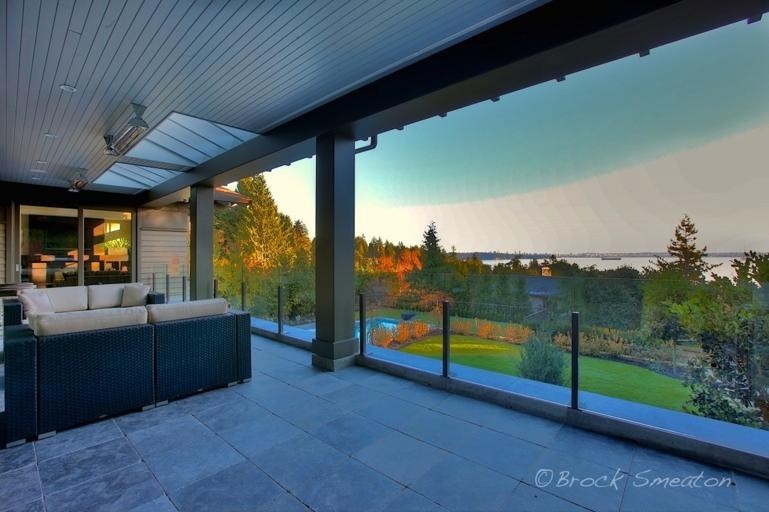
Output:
[68,168,89,192]
[104,103,149,156]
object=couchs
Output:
[0,271,252,448]
